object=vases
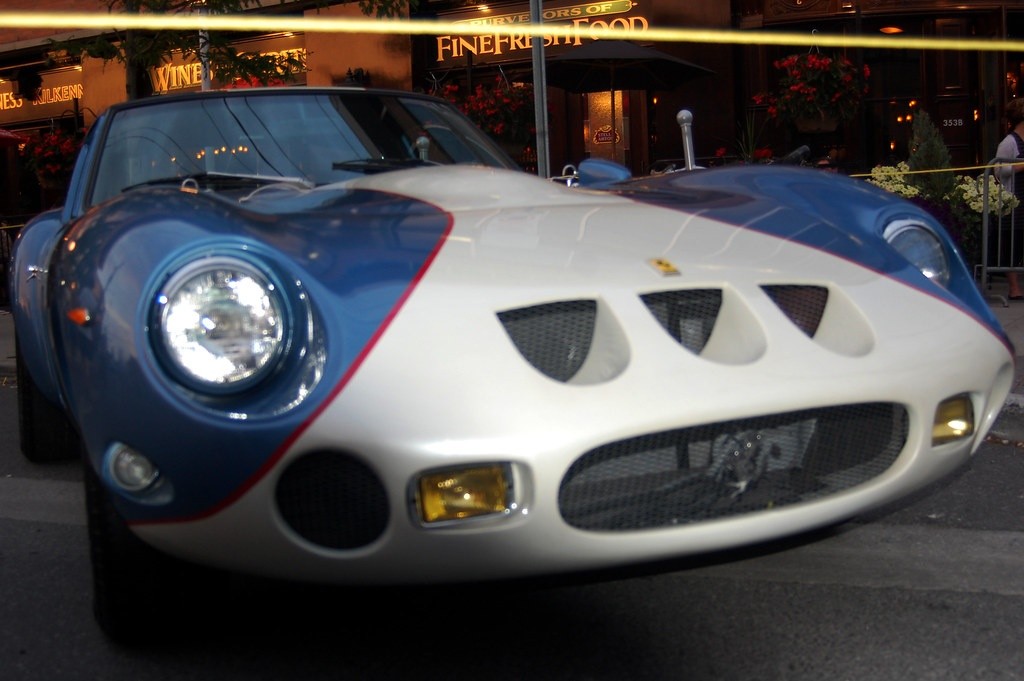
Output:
[34,170,71,190]
[793,112,840,134]
[497,141,524,156]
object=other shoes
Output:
[1008,293,1024,300]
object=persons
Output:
[995,97,1024,300]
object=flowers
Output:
[22,128,85,175]
[421,82,466,114]
[750,54,871,115]
[468,73,538,138]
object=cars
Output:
[9,84,1016,644]
